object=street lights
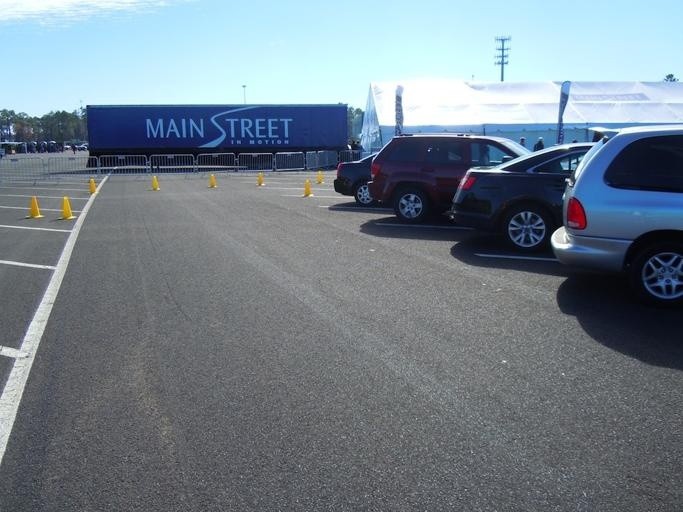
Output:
[241,84,247,103]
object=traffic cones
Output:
[152,176,160,191]
[57,195,77,219]
[208,174,218,188]
[256,172,264,186]
[304,178,314,197]
[89,176,98,194]
[316,170,325,184]
[26,196,45,218]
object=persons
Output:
[519,137,526,146]
[533,137,544,152]
